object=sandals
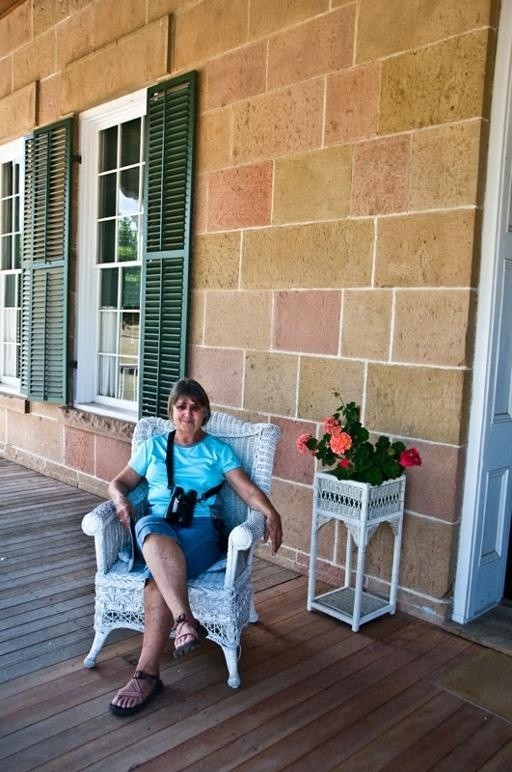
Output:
[109,672,163,717]
[171,613,209,658]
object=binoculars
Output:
[163,486,197,527]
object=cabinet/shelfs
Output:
[304,470,408,632]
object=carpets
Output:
[428,647,512,722]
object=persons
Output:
[107,376,283,717]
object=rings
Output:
[119,518,125,522]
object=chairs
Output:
[78,408,279,692]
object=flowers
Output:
[296,388,422,485]
